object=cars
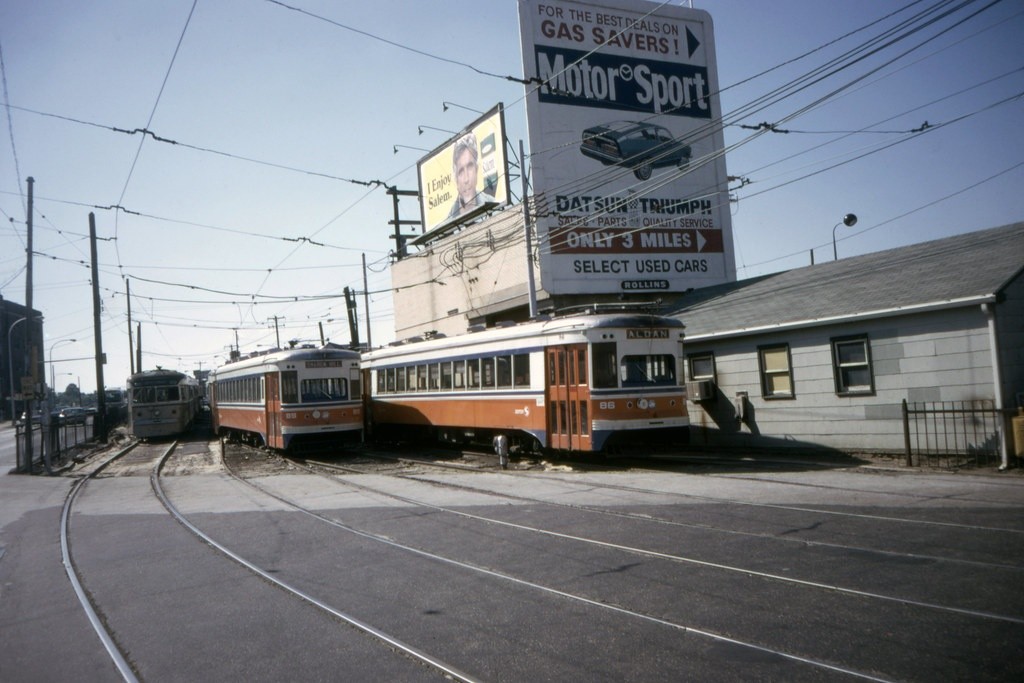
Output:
[20,410,44,425]
[59,408,90,425]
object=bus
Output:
[206,348,366,457]
[365,299,691,467]
[124,366,203,440]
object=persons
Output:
[447,131,498,220]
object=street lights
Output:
[50,339,77,390]
[9,315,45,429]
